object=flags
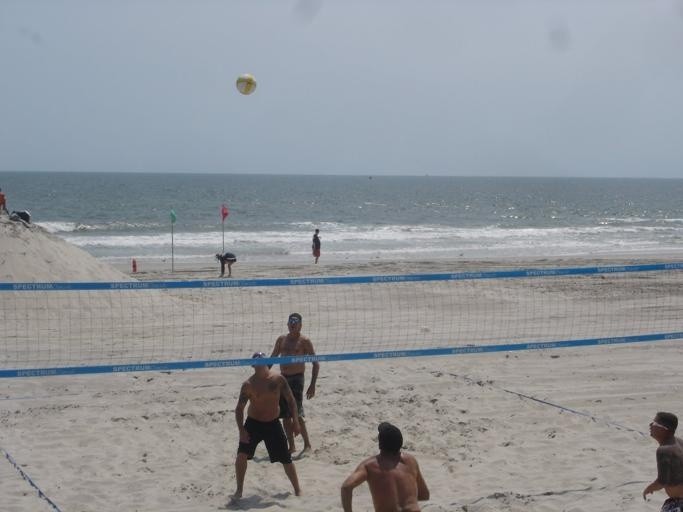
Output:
[219,205,230,222]
[168,209,177,225]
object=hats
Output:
[378,422,403,449]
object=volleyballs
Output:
[236,74,256,95]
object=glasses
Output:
[651,420,668,429]
[288,317,300,325]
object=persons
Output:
[264,310,321,458]
[311,228,322,264]
[225,350,304,502]
[340,421,430,512]
[216,252,237,279]
[0,188,7,212]
[9,209,31,228]
[640,410,683,512]
